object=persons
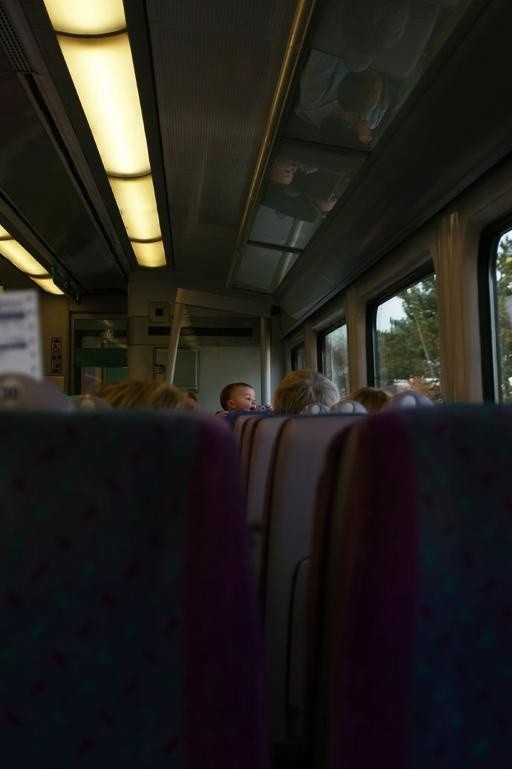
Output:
[0,367,436,417]
